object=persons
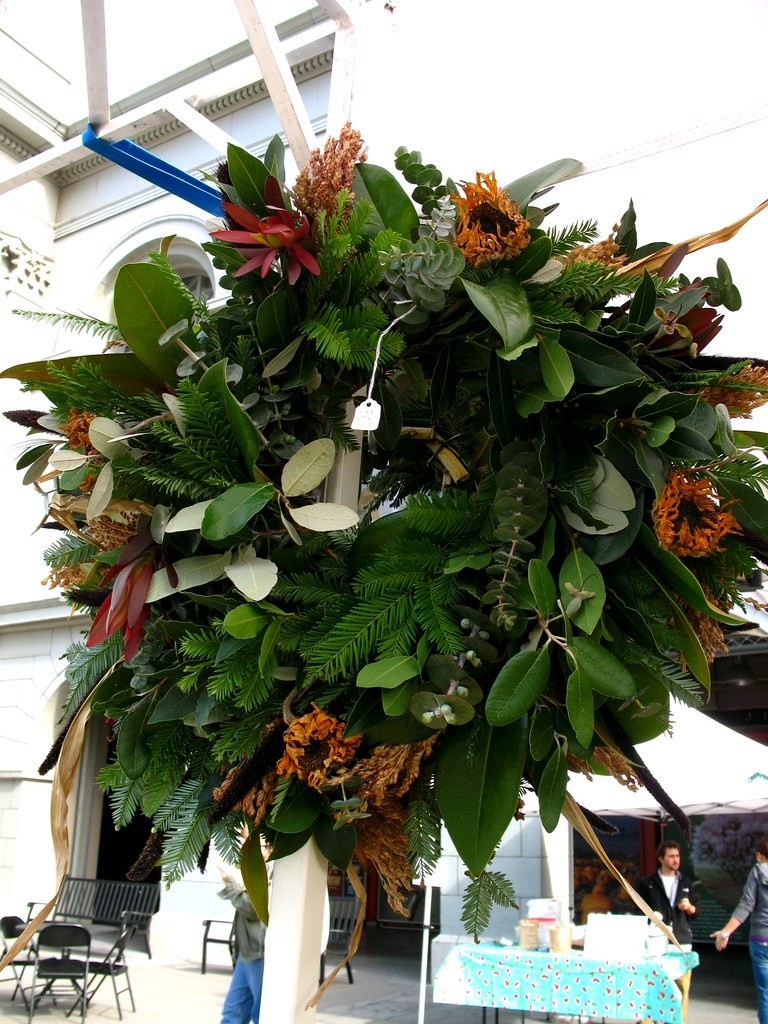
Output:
[636,841,701,1024]
[709,836,768,1024]
[208,827,274,1024]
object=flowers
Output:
[1,111,768,1004]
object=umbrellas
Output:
[511,692,768,817]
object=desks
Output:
[434,942,700,1024]
[14,922,118,1010]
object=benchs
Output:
[26,875,160,962]
[202,892,358,987]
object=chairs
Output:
[66,924,135,1021]
[0,916,57,1012]
[28,924,92,1024]
